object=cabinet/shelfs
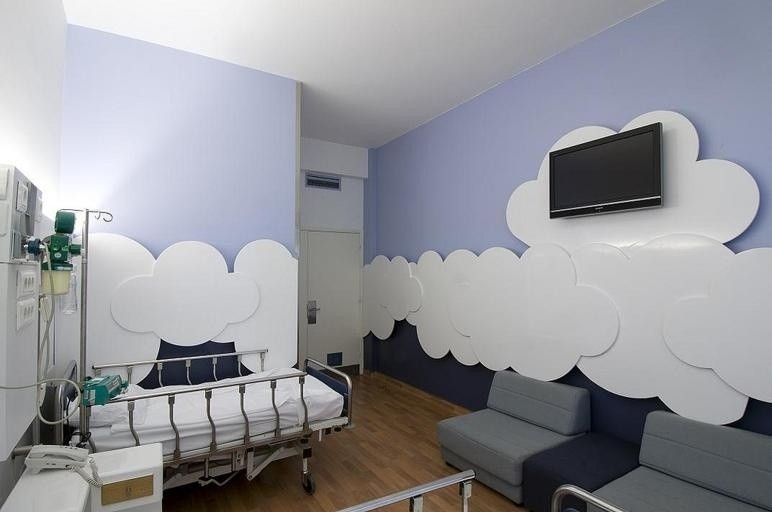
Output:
[0,443,164,512]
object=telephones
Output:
[24,444,88,475]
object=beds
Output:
[54,349,352,496]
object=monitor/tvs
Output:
[549,122,662,219]
